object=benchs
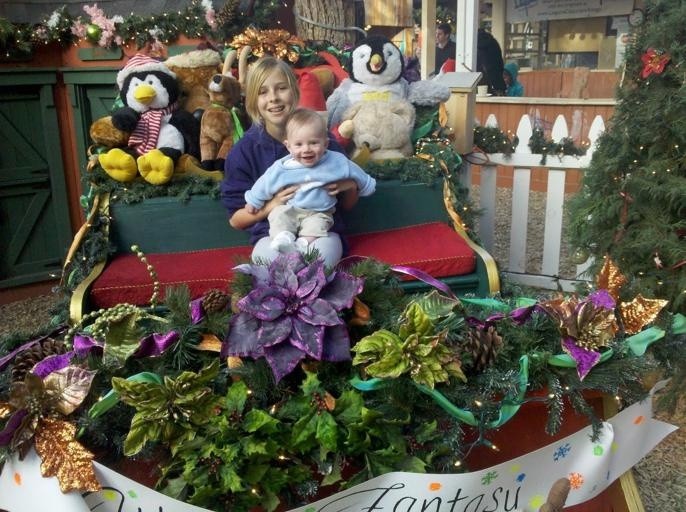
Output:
[58,153,503,330]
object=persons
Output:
[502,61,526,97]
[434,22,456,73]
[477,28,508,97]
[219,57,360,276]
[243,107,378,252]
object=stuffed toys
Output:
[337,96,417,161]
[196,71,244,171]
[97,51,200,187]
[324,36,453,145]
[88,45,227,180]
